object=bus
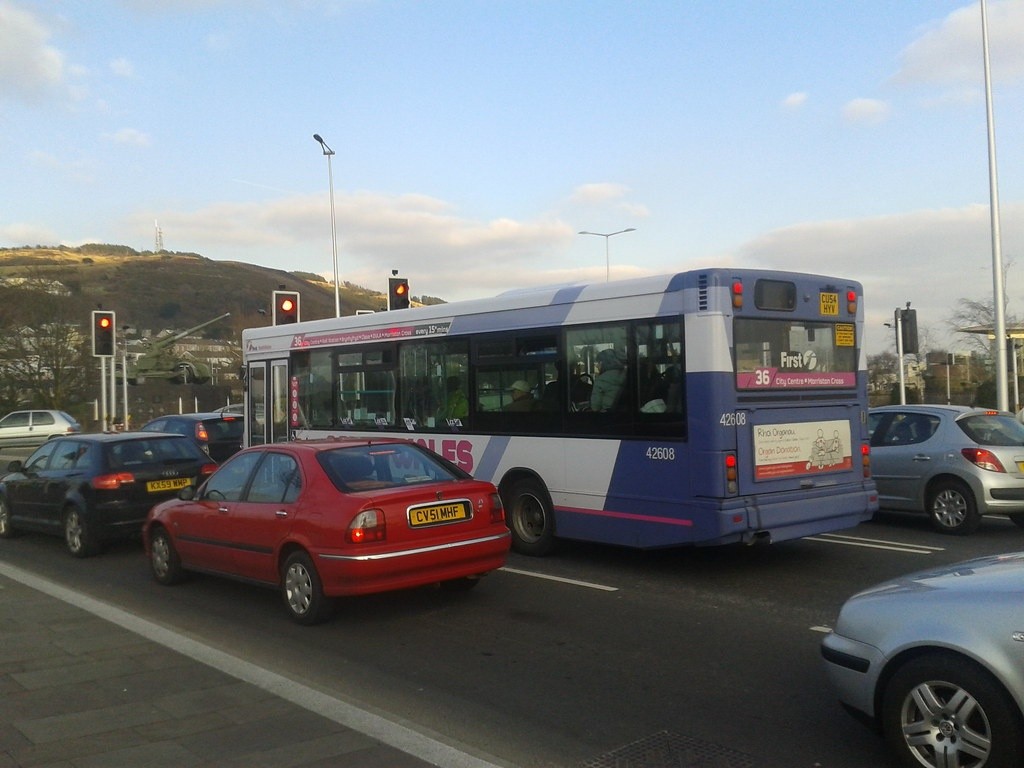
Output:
[240,268,880,562]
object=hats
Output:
[505,380,531,393]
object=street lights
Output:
[312,133,341,318]
[579,227,636,285]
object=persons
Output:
[501,346,684,415]
[403,372,470,422]
[914,420,932,441]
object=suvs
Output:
[0,430,221,558]
[138,411,263,465]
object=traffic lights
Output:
[274,293,299,324]
[389,279,410,312]
[93,312,116,357]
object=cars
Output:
[864,403,1024,537]
[140,436,513,626]
[820,545,1024,768]
[0,409,82,446]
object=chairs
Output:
[546,368,669,412]
[335,455,375,483]
[894,419,933,443]
[120,441,145,462]
